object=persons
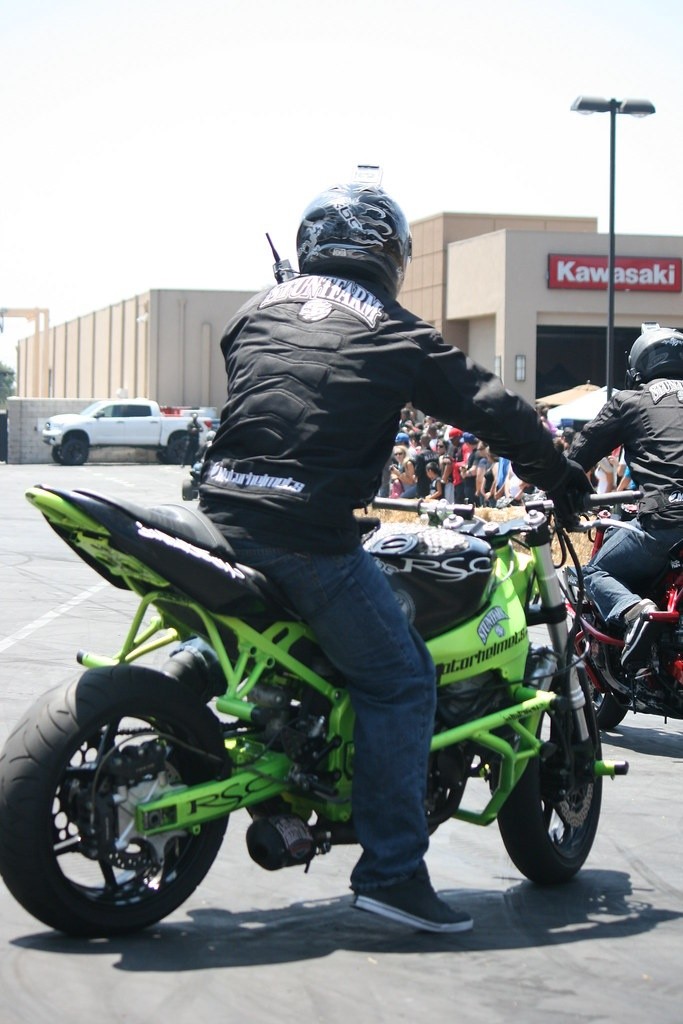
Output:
[195,188,596,931]
[181,412,217,469]
[567,328,683,667]
[388,407,636,511]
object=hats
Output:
[563,427,575,437]
[464,433,478,444]
[395,432,409,443]
[449,428,463,440]
[459,432,469,443]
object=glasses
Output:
[436,445,446,448]
[424,421,428,423]
[394,452,403,456]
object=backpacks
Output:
[450,456,463,486]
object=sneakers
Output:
[617,597,659,668]
[351,858,474,935]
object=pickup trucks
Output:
[43,397,211,466]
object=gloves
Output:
[511,441,598,528]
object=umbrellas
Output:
[535,380,602,404]
[547,386,621,420]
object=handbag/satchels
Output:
[400,473,418,498]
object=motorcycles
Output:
[547,504,683,731]
[0,482,658,937]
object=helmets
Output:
[296,181,412,298]
[627,328,683,381]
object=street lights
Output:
[571,96,656,400]
